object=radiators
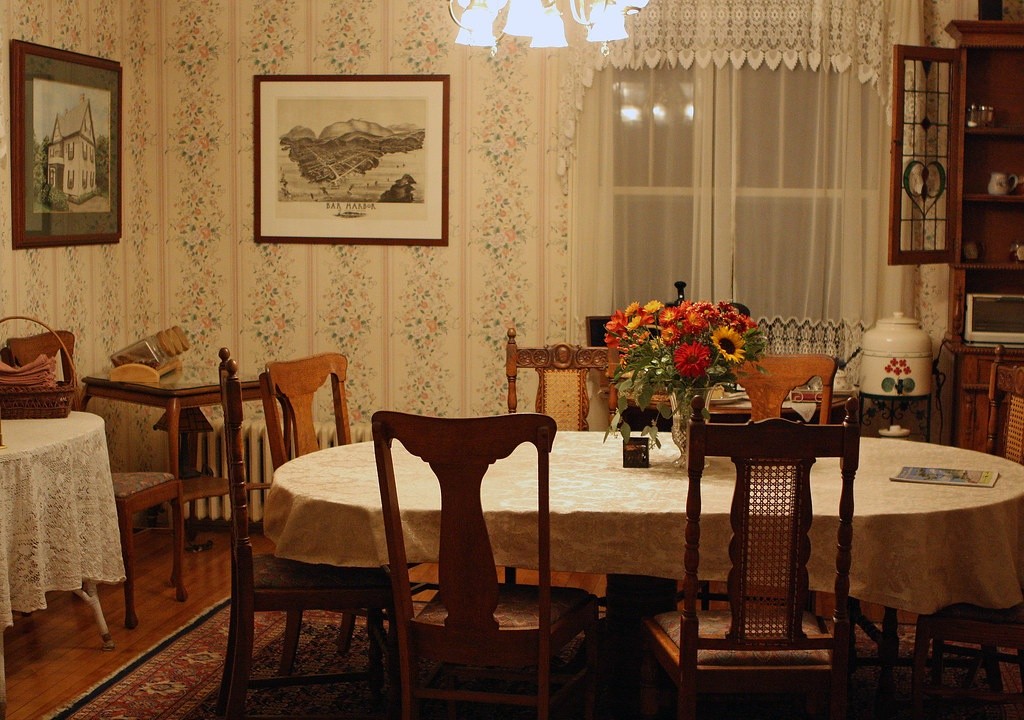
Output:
[172,416,375,523]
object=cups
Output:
[988,172,1019,195]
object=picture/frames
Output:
[585,315,611,346]
[9,37,129,249]
[254,72,453,249]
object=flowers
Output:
[597,302,757,402]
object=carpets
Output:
[34,589,1024,720]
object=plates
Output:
[833,390,853,395]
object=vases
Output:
[668,378,713,472]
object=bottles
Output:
[1009,238,1024,263]
[833,369,849,390]
[110,324,190,369]
[970,104,992,127]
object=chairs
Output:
[215,329,1024,720]
[7,330,184,630]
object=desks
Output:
[1,410,127,654]
[82,363,275,601]
[262,432,1024,720]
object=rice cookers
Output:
[859,312,932,396]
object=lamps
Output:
[446,0,651,61]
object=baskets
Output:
[0,315,78,420]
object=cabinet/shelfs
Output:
[886,17,1024,446]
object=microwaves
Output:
[965,294,1024,343]
